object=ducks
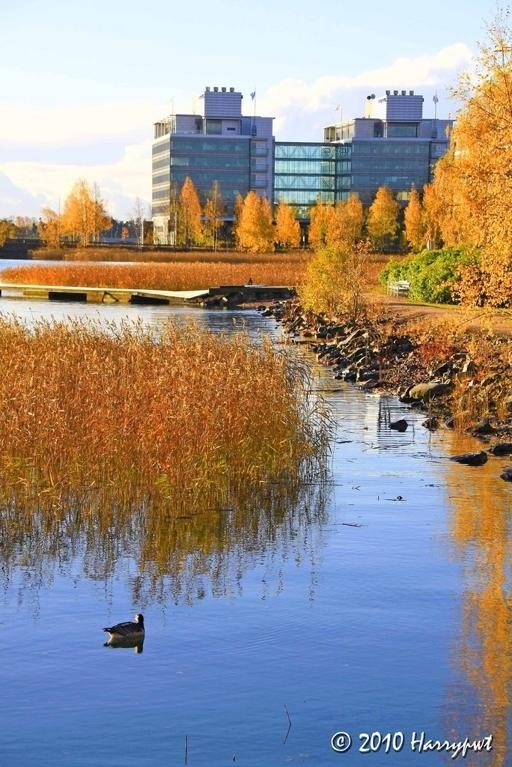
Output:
[101,613,144,639]
